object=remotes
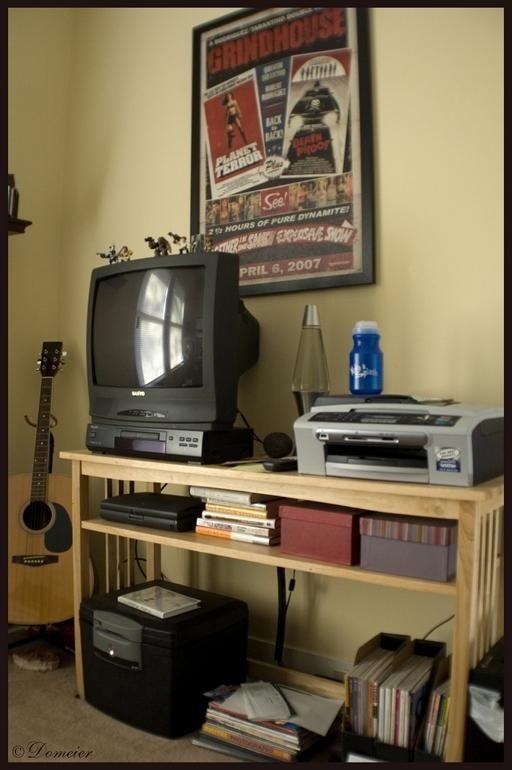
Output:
[263,455,297,472]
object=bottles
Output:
[348,319,385,396]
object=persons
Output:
[220,92,249,158]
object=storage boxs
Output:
[279,499,359,566]
[357,512,456,583]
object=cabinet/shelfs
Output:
[58,447,508,763]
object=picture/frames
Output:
[189,8,375,297]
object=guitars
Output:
[8,342,97,625]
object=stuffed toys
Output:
[97,232,214,264]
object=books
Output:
[189,486,289,546]
[192,647,451,762]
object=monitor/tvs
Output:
[85,251,262,430]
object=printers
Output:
[292,394,505,487]
